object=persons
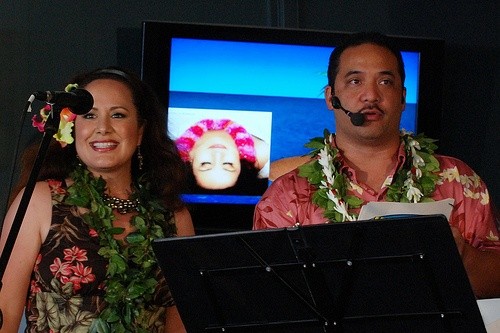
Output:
[0,67,197,333]
[251,35,500,301]
[168,107,272,197]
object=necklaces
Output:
[68,162,178,333]
[292,127,441,223]
[101,190,139,214]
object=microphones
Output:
[32,88,94,115]
[332,95,365,126]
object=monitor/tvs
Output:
[138,19,448,231]
